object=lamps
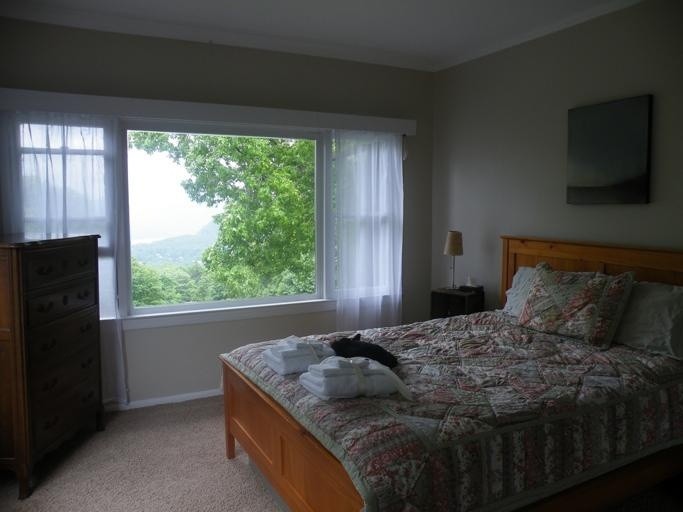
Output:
[443,231,464,291]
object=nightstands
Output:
[431,287,484,320]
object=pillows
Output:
[502,261,682,362]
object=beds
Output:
[218,235,681,511]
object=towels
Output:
[263,338,397,399]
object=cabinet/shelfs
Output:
[1,235,108,500]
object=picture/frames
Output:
[566,95,653,204]
